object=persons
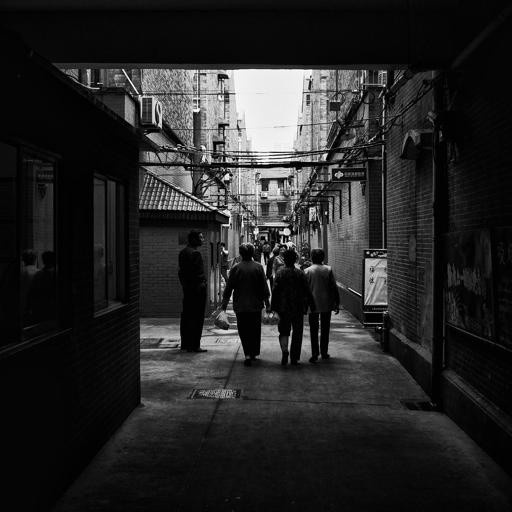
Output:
[269,248,308,367]
[302,248,340,362]
[178,228,209,353]
[218,241,272,362]
[220,237,311,296]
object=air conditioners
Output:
[140,96,162,131]
[261,192,268,198]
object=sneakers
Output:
[188,350,207,353]
[308,356,319,363]
[251,354,257,359]
[291,359,298,367]
[244,353,249,357]
[180,346,188,351]
[322,354,330,358]
[281,352,289,365]
